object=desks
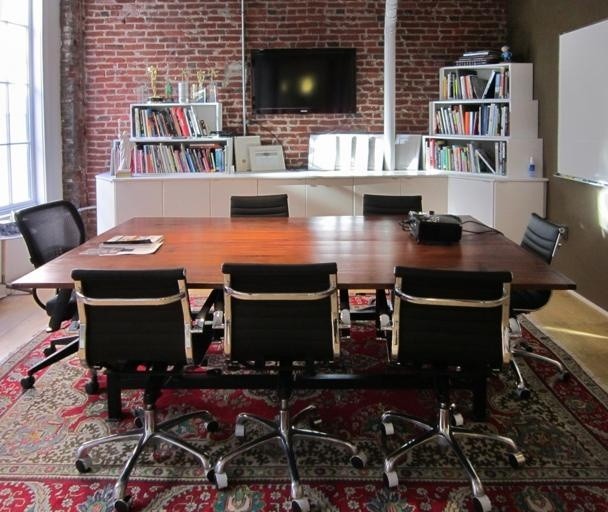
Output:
[4,215,578,423]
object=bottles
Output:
[110,139,122,175]
[528,157,536,177]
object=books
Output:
[109,109,229,176]
[424,72,510,177]
[454,50,499,65]
[78,235,164,256]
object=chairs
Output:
[228,193,290,219]
[348,192,423,342]
[504,211,570,402]
[376,265,527,511]
[12,199,100,394]
[70,268,219,512]
[213,263,368,512]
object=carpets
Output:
[1,293,607,512]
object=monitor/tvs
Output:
[251,48,356,114]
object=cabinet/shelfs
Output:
[420,61,544,176]
[95,176,209,238]
[400,176,446,214]
[127,102,235,172]
[446,175,546,247]
[306,177,400,215]
[209,177,306,217]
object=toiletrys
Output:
[527,157,537,179]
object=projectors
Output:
[409,213,462,245]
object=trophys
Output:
[146,64,220,103]
